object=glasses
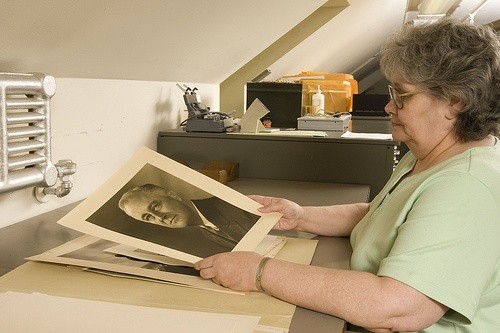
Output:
[388,84,442,109]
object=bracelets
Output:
[256,257,271,293]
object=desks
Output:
[0,178,371,333]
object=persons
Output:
[118,184,261,252]
[193,16,500,333]
